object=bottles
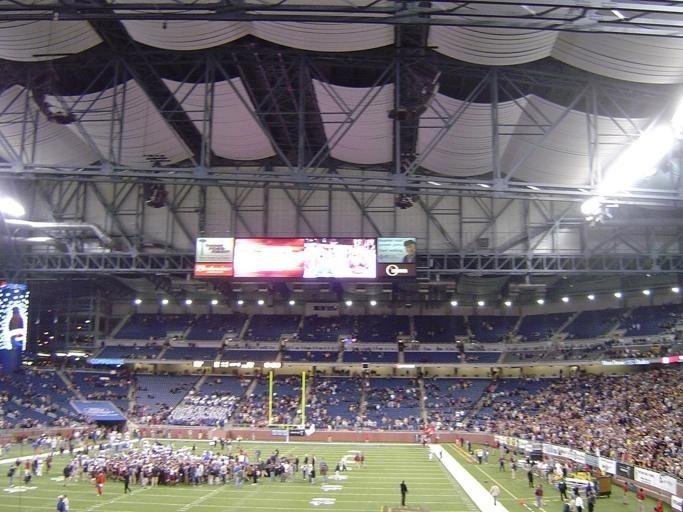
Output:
[9,306,24,350]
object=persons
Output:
[0,304,683,512]
[402,239,415,264]
